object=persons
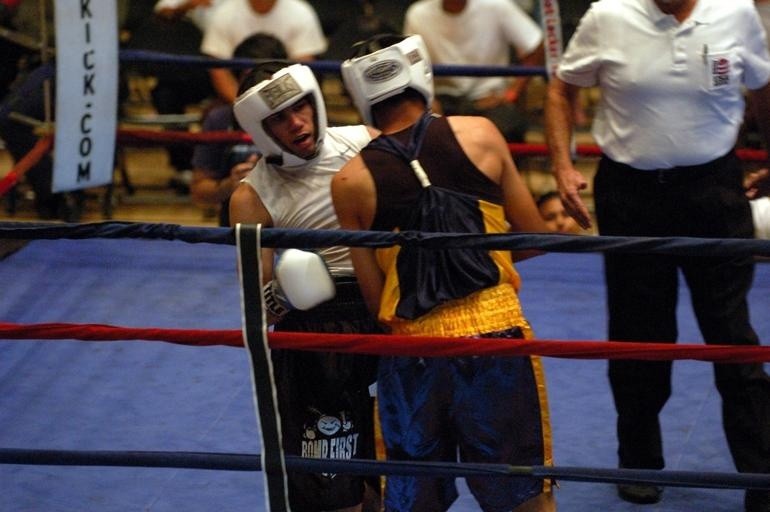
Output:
[123,1,581,239]
[227,59,383,510]
[540,0,769,510]
[327,31,560,510]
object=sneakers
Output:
[618,462,660,503]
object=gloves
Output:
[262,248,335,317]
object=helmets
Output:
[342,34,435,129]
[233,62,327,170]
